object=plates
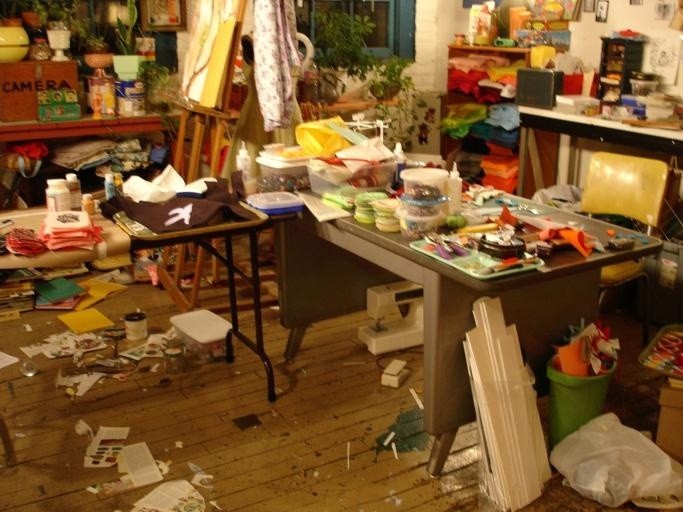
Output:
[351,192,402,233]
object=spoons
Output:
[425,232,469,259]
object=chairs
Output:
[568,150,674,347]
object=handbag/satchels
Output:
[564,66,583,95]
[3,141,48,160]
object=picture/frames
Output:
[582,0,595,12]
[139,0,186,33]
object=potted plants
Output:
[45,0,78,61]
[305,9,374,106]
[17,0,44,29]
[110,1,141,82]
[80,35,113,79]
[133,20,156,63]
[367,53,438,154]
[0,0,30,65]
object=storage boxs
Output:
[303,158,398,197]
[254,147,309,193]
[653,387,681,464]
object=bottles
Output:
[81,194,96,215]
[103,173,124,199]
[45,179,69,212]
[393,141,408,187]
[303,58,320,101]
[122,311,148,344]
[235,142,251,181]
[453,34,465,47]
[447,162,462,212]
[161,347,186,375]
[65,173,82,212]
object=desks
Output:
[264,188,665,477]
[512,104,683,232]
[0,197,277,468]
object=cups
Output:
[18,358,40,377]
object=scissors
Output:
[106,372,130,382]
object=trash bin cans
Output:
[546,357,618,448]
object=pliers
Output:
[424,236,468,261]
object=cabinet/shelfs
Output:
[444,42,561,201]
